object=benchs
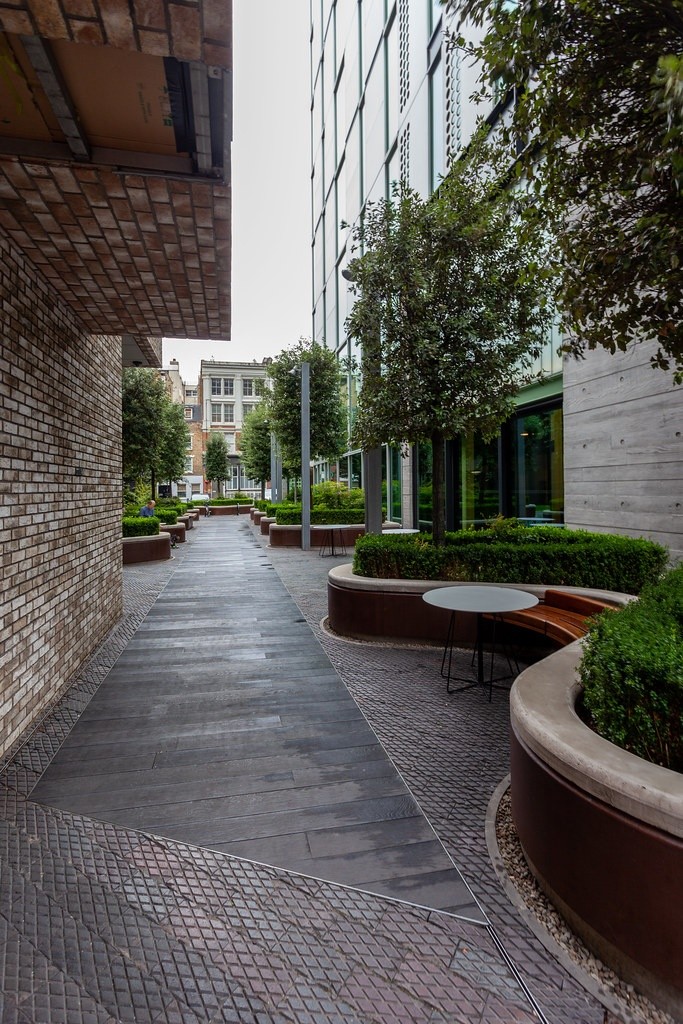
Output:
[481,590,620,646]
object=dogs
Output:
[170,534,180,548]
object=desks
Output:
[313,525,350,558]
[380,529,420,534]
[518,517,564,527]
[422,586,539,702]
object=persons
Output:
[140,500,156,517]
